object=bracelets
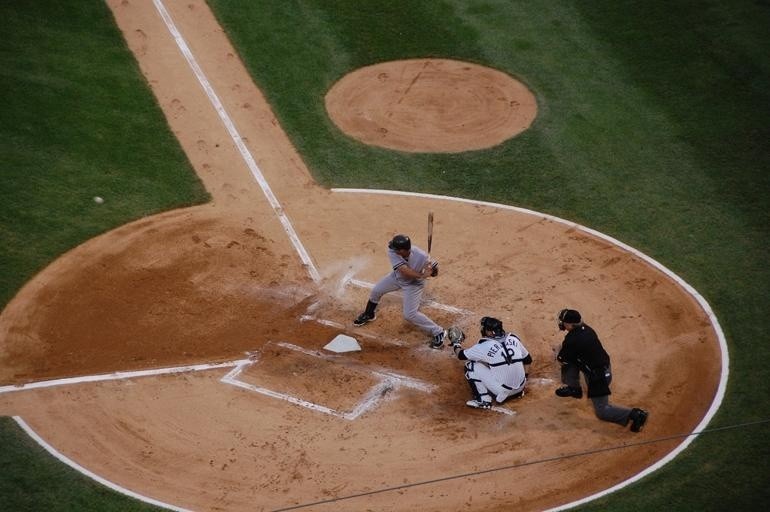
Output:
[525,373,529,378]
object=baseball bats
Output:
[427,211,433,279]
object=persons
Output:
[554,308,649,433]
[353,234,447,349]
[448,316,533,411]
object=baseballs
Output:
[95,196,103,203]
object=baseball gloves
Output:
[448,326,465,346]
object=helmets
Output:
[558,309,581,329]
[389,235,411,249]
[481,317,503,337]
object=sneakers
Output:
[354,312,376,326]
[556,386,582,398]
[466,400,491,409]
[631,409,647,431]
[431,330,447,348]
